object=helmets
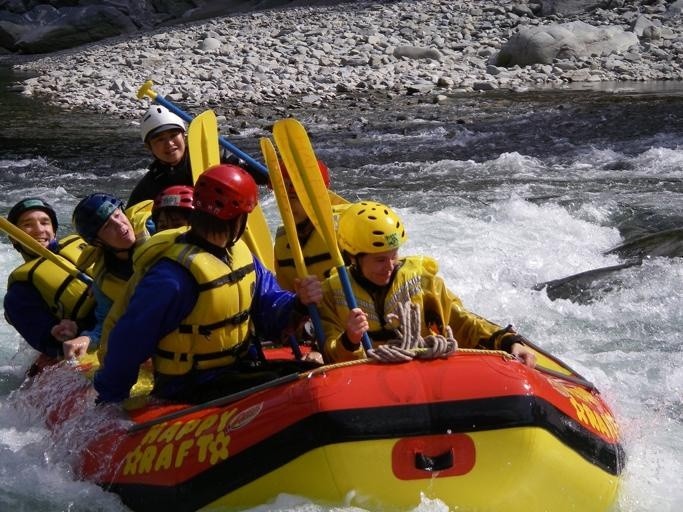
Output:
[72,191,125,246]
[337,200,411,257]
[139,102,187,142]
[151,184,195,224]
[266,159,331,200]
[6,197,59,248]
[193,163,259,220]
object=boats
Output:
[27,328,626,512]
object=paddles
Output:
[239,200,307,375]
[187,110,220,189]
[272,118,374,351]
[136,81,351,205]
[259,136,330,351]
[1,217,93,285]
